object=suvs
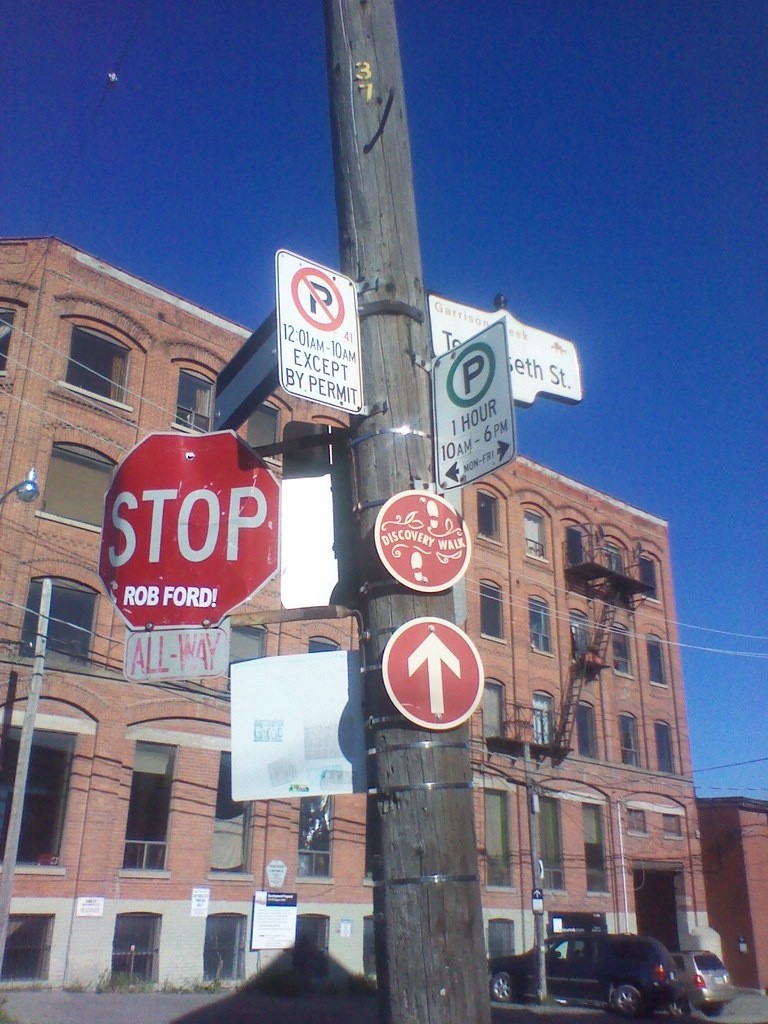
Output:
[668,949,736,1024]
[488,931,681,1022]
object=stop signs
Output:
[95,427,282,634]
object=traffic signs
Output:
[430,315,519,495]
